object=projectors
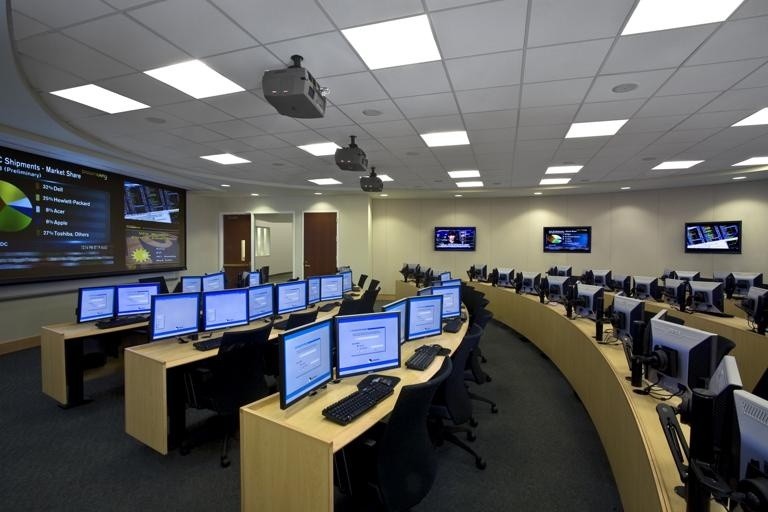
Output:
[359,178,384,193]
[262,69,327,118]
[335,146,368,172]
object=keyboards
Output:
[316,304,338,311]
[194,336,224,352]
[321,382,393,425]
[272,320,287,328]
[405,345,439,371]
[443,318,463,332]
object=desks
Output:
[240,281,469,512]
[464,280,731,512]
[601,291,764,400]
[122,284,367,457]
[42,314,150,406]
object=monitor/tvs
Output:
[419,268,431,289]
[262,267,269,285]
[280,317,333,409]
[649,317,717,405]
[79,286,116,325]
[554,264,572,276]
[338,271,353,295]
[612,295,644,345]
[201,272,224,290]
[723,389,764,512]
[685,221,744,256]
[306,276,321,308]
[707,356,742,438]
[204,289,249,332]
[665,277,687,312]
[493,267,513,288]
[443,280,462,286]
[321,275,342,302]
[648,310,669,362]
[153,295,200,338]
[277,283,307,315]
[400,262,418,287]
[545,274,570,311]
[590,269,612,293]
[440,272,450,281]
[689,281,725,317]
[137,277,169,293]
[179,276,202,293]
[334,313,401,378]
[250,288,274,323]
[518,270,541,300]
[610,268,630,297]
[119,286,159,319]
[408,295,443,343]
[745,286,764,335]
[674,269,700,301]
[246,273,259,286]
[429,286,460,323]
[434,227,477,252]
[568,263,587,284]
[471,265,487,281]
[630,275,656,302]
[543,227,592,253]
[575,282,604,324]
[384,298,407,347]
[729,269,762,300]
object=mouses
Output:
[433,344,441,351]
[380,379,392,385]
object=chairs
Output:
[188,316,275,467]
[469,309,493,329]
[357,274,368,288]
[286,306,319,331]
[469,299,489,315]
[467,291,485,311]
[441,323,499,442]
[336,355,454,512]
[339,298,365,316]
[644,311,659,321]
[368,279,380,290]
[361,287,382,314]
[717,335,734,360]
[665,314,684,325]
[463,285,475,304]
[461,282,467,299]
